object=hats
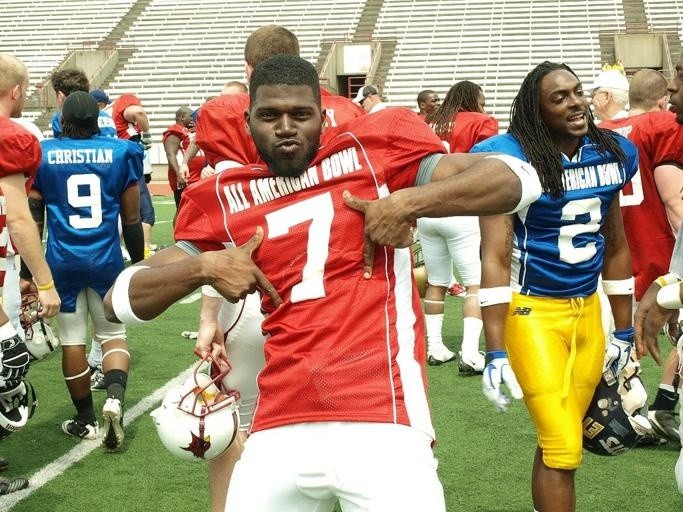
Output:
[580,74,627,94]
[351,85,377,104]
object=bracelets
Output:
[477,286,512,307]
[38,280,54,291]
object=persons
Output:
[417,90,467,297]
[193,29,365,512]
[352,86,384,115]
[0,54,61,494]
[480,61,639,512]
[587,70,630,348]
[634,59,683,490]
[599,69,683,444]
[19,92,145,450]
[163,81,248,229]
[51,69,116,390]
[416,81,499,376]
[93,91,157,259]
[104,55,541,512]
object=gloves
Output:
[602,326,635,380]
[481,352,524,413]
[0,320,30,381]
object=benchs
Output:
[0,1,365,139]
[371,3,682,134]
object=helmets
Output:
[18,292,60,366]
[0,378,34,437]
[582,360,651,457]
[149,353,240,463]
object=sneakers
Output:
[100,396,125,452]
[645,403,681,443]
[0,475,28,496]
[61,418,99,441]
[85,352,108,391]
[456,350,484,375]
[425,347,457,365]
